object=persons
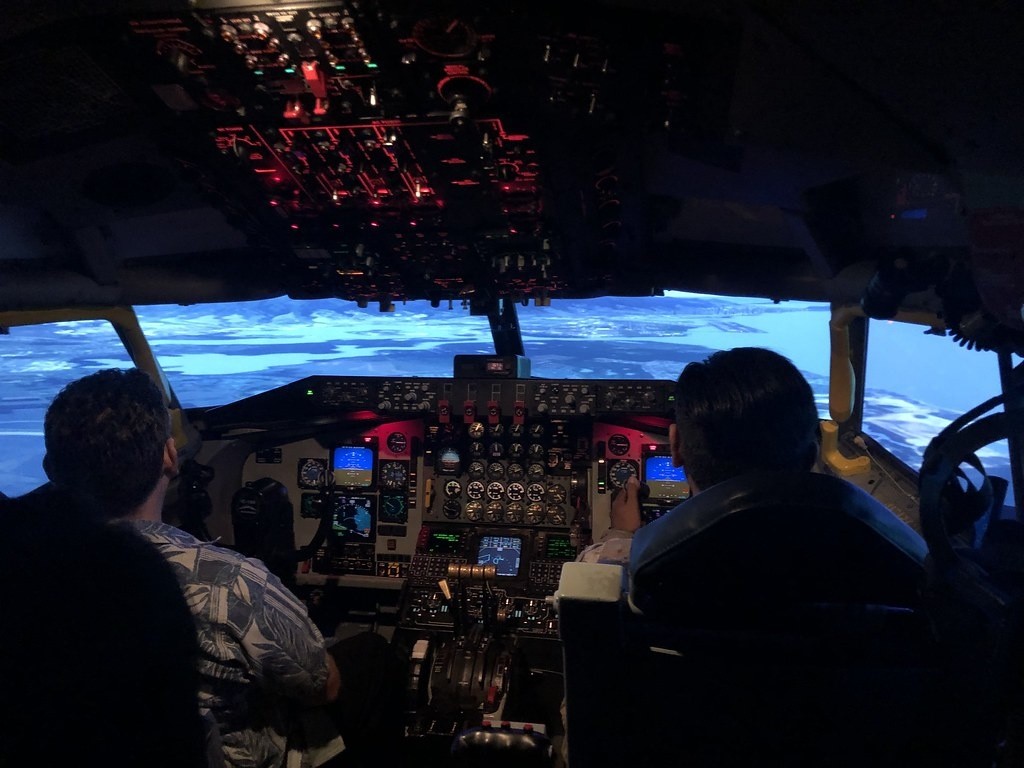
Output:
[44,369,345,767]
[554,346,823,766]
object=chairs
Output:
[556,473,1005,767]
[0,500,225,768]
